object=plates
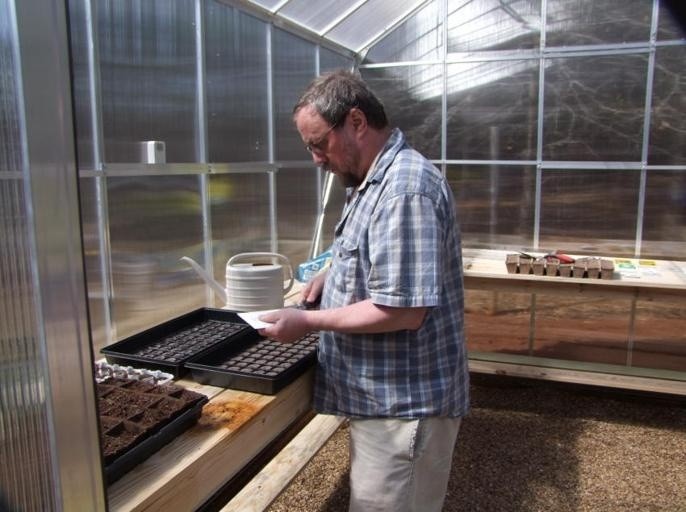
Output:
[99,307,251,381]
[184,326,319,395]
[103,396,208,488]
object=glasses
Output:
[305,104,360,155]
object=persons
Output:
[255,70,471,512]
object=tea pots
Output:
[179,253,294,313]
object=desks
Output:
[108,372,317,511]
[459,255,686,398]
